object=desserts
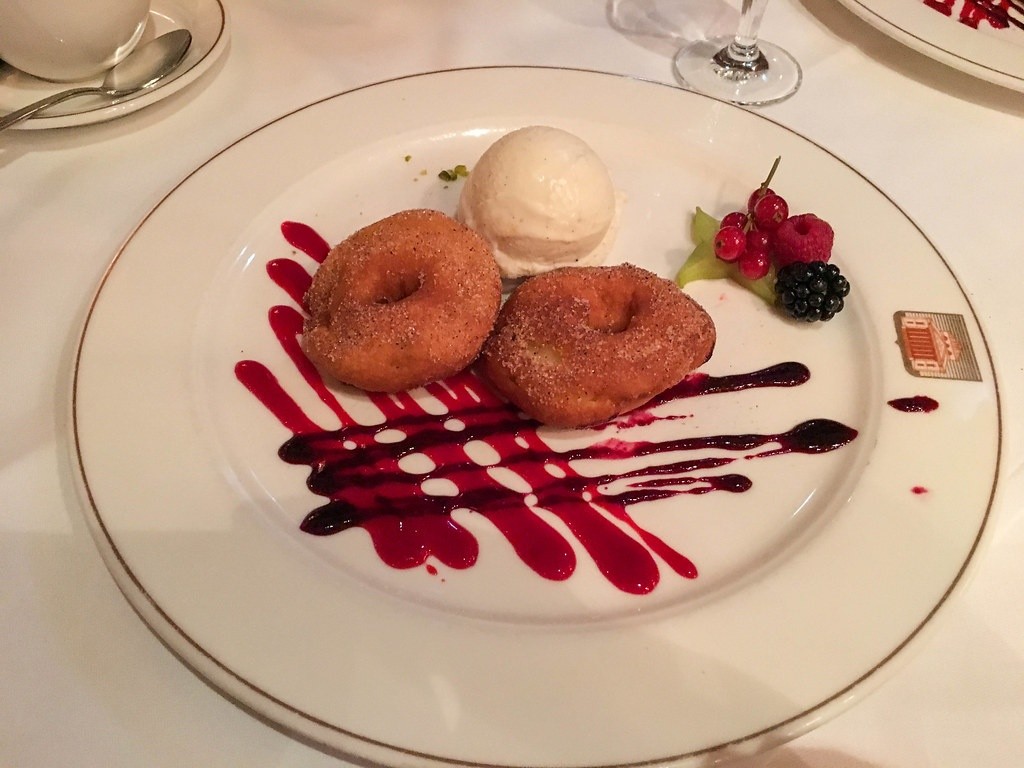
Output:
[455,125,614,279]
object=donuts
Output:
[480,265,717,428]
[302,207,501,390]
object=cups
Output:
[0,0,152,85]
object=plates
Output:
[837,0,1024,93]
[0,0,231,130]
[73,66,1002,768]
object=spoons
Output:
[0,29,192,130]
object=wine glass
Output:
[675,0,803,104]
